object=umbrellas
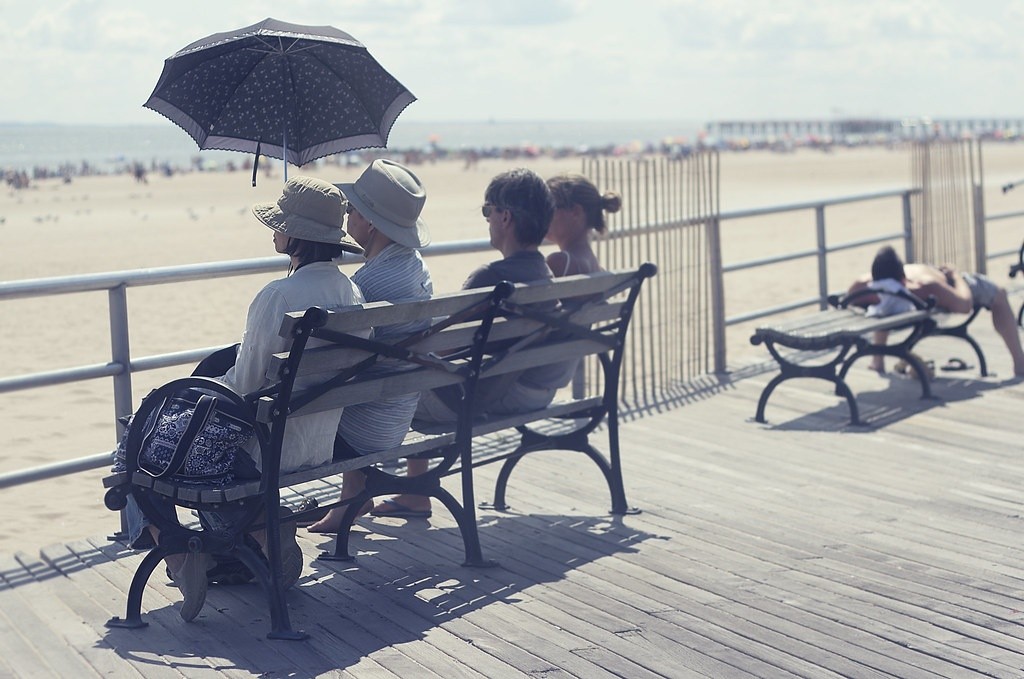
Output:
[141,16,418,186]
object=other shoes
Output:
[266,505,303,590]
[206,560,254,585]
[294,496,322,527]
[165,551,212,622]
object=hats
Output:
[251,175,347,244]
[331,158,432,249]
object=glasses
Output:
[346,201,354,214]
[481,201,504,217]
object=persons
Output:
[371,171,620,519]
[165,158,433,584]
[842,246,1024,380]
[124,174,375,621]
[306,168,565,533]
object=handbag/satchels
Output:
[111,395,258,487]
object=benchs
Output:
[750,284,991,426]
[103,263,656,641]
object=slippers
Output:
[939,356,974,371]
[369,497,432,517]
